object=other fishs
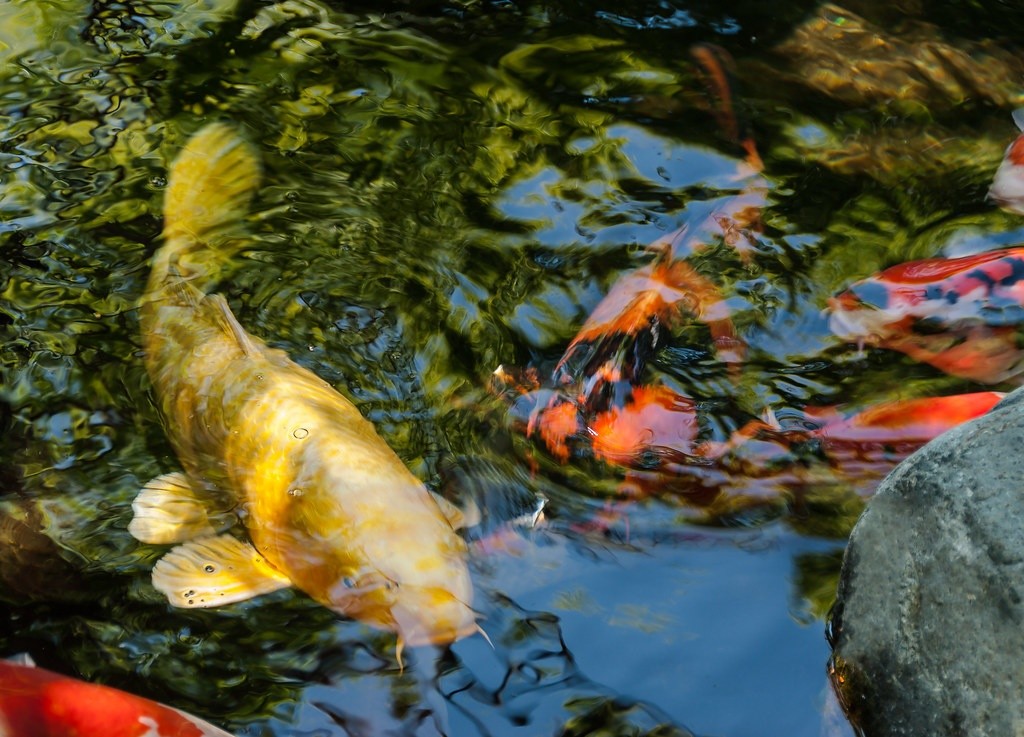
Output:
[126,120,497,672]
[465,43,1023,602]
[0,652,242,736]
